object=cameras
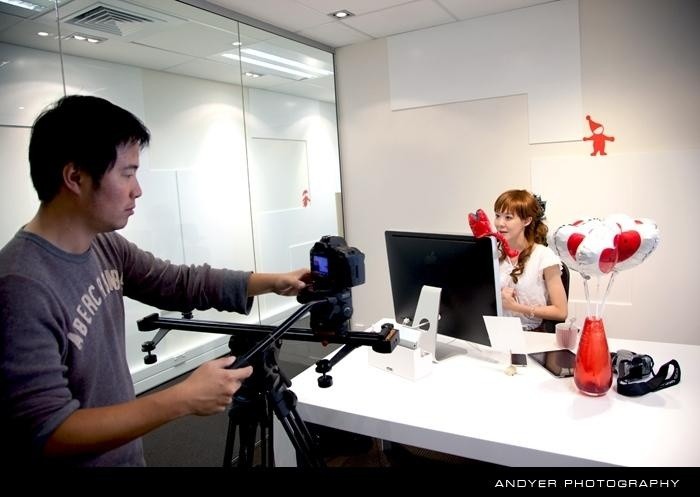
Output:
[309,236,365,289]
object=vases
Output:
[573,316,614,398]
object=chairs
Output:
[528,261,570,334]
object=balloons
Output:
[546,217,662,279]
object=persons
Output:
[494,189,568,366]
[0,95,312,467]
[468,209,519,258]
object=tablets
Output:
[528,349,576,378]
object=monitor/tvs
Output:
[385,230,503,364]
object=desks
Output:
[282,317,700,467]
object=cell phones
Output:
[511,354,527,367]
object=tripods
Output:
[222,335,327,467]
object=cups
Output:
[555,323,578,350]
